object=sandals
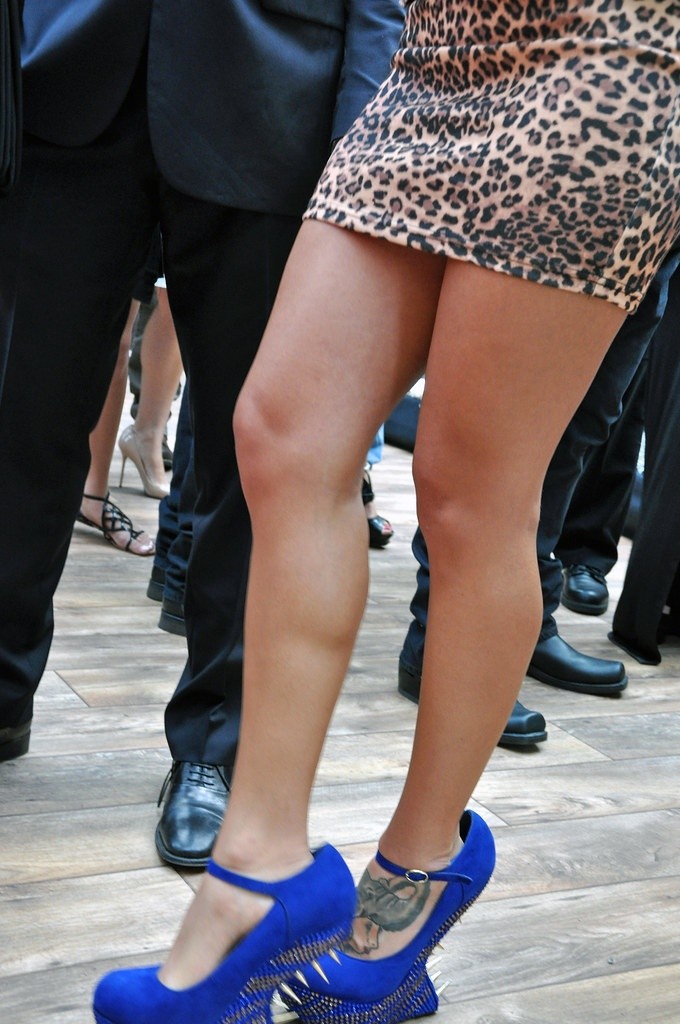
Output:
[76,491,156,554]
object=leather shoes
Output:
[527,634,628,694]
[0,719,31,763]
[398,656,547,744]
[561,565,609,616]
[155,761,234,867]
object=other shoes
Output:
[130,403,173,466]
[147,565,165,601]
[158,599,188,637]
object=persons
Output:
[0,0,680,1024]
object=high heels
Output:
[93,844,355,1024]
[277,810,496,1024]
[362,478,393,546]
[119,425,170,499]
